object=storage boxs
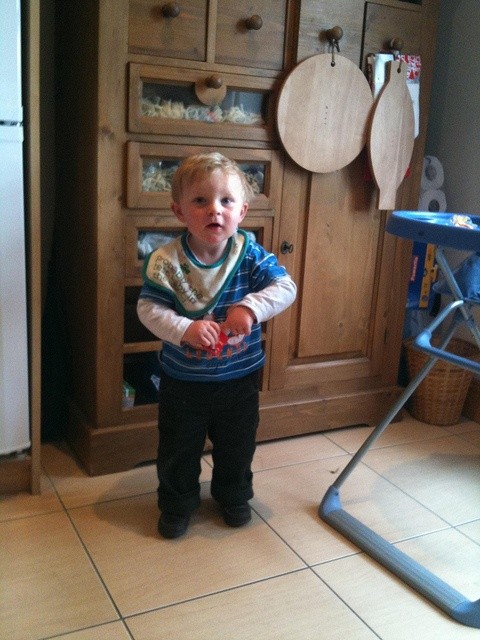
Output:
[406,241,435,308]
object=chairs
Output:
[318,211,480,628]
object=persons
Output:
[137,151,297,538]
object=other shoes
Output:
[158,510,190,539]
[215,498,252,526]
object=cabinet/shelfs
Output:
[49,0,438,475]
